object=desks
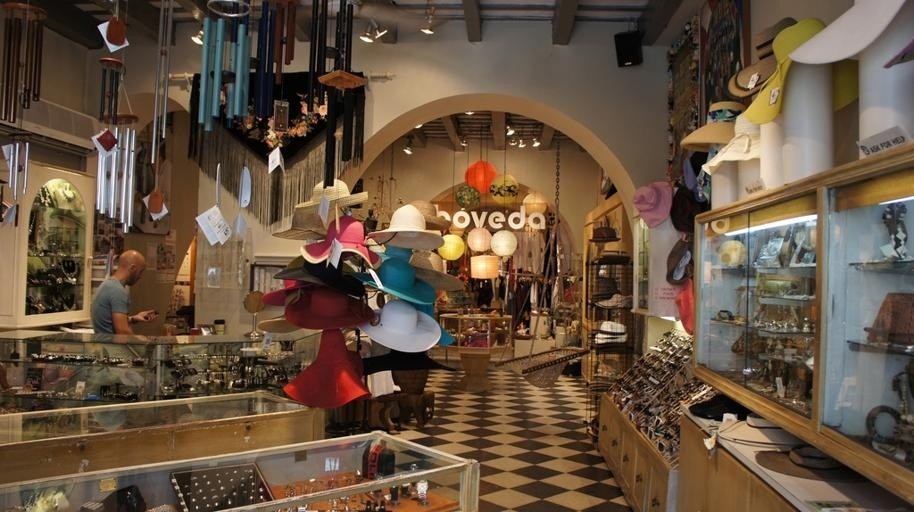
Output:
[439,313,514,364]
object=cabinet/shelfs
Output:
[1,158,98,330]
[676,404,911,511]
[1,328,325,484]
[598,328,741,511]
[693,141,913,505]
[0,430,481,511]
[585,250,634,444]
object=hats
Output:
[595,320,628,344]
[586,359,625,440]
[681,0,908,176]
[666,239,695,335]
[633,181,673,228]
[588,227,621,242]
[257,179,463,410]
[592,277,632,309]
[689,393,859,483]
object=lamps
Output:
[400,135,416,157]
[186,4,213,48]
[437,122,548,286]
[357,13,391,52]
[417,3,442,39]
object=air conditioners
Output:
[1,82,107,156]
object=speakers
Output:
[613,29,643,68]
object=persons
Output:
[88,249,158,336]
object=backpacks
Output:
[670,181,708,233]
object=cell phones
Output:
[144,310,159,320]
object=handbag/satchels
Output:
[731,334,767,362]
